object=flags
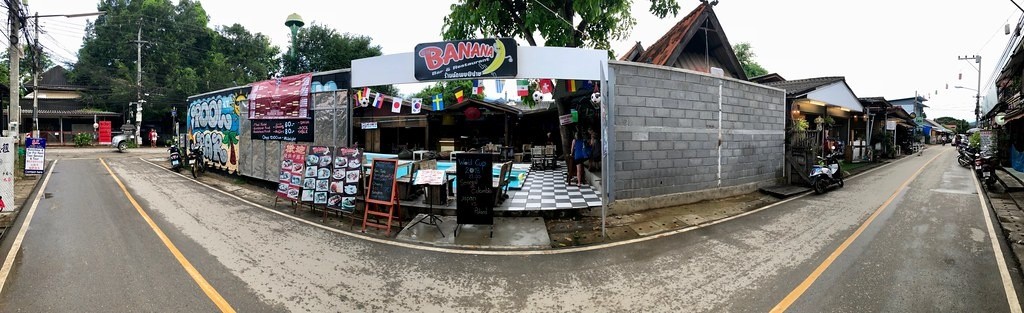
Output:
[391,97,403,114]
[453,85,464,103]
[353,91,362,107]
[565,80,577,92]
[432,93,444,111]
[372,92,385,109]
[472,79,483,95]
[540,79,554,94]
[362,87,371,99]
[411,98,423,114]
[516,80,528,96]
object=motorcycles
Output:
[165,139,185,173]
[187,143,206,178]
[957,140,999,191]
[808,145,844,195]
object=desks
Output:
[362,160,412,198]
[500,147,512,162]
[445,164,456,207]
[450,151,466,163]
[413,150,429,161]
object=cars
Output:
[951,134,968,147]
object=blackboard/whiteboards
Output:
[364,157,397,206]
[455,152,494,225]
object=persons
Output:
[545,132,554,146]
[148,128,159,148]
[569,125,601,187]
[955,132,962,149]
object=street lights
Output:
[9,9,106,131]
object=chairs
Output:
[396,162,419,202]
[493,164,508,207]
[359,155,371,196]
[503,161,513,196]
[481,144,557,171]
[401,159,426,195]
[420,161,449,205]
[428,159,455,197]
